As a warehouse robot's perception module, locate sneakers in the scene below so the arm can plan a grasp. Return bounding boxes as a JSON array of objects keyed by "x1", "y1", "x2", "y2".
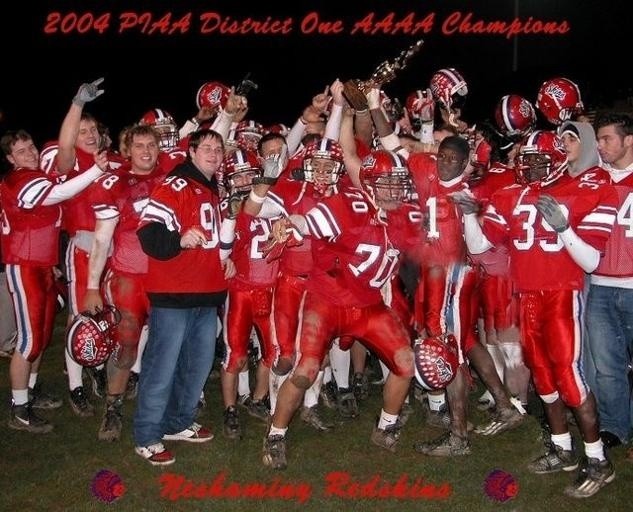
[
  {"x1": 29, "y1": 383, "x2": 63, "y2": 409},
  {"x1": 85, "y1": 367, "x2": 107, "y2": 398},
  {"x1": 337, "y1": 388, "x2": 359, "y2": 418},
  {"x1": 301, "y1": 404, "x2": 333, "y2": 433},
  {"x1": 414, "y1": 432, "x2": 473, "y2": 456},
  {"x1": 162, "y1": 423, "x2": 214, "y2": 443},
  {"x1": 425, "y1": 403, "x2": 474, "y2": 433},
  {"x1": 9, "y1": 399, "x2": 53, "y2": 433},
  {"x1": 126, "y1": 372, "x2": 140, "y2": 400},
  {"x1": 237, "y1": 394, "x2": 253, "y2": 409},
  {"x1": 68, "y1": 387, "x2": 94, "y2": 417},
  {"x1": 475, "y1": 407, "x2": 526, "y2": 439},
  {"x1": 369, "y1": 417, "x2": 404, "y2": 453},
  {"x1": 321, "y1": 383, "x2": 338, "y2": 409},
  {"x1": 196, "y1": 399, "x2": 208, "y2": 417},
  {"x1": 135, "y1": 443, "x2": 176, "y2": 465},
  {"x1": 248, "y1": 401, "x2": 272, "y2": 420},
  {"x1": 265, "y1": 415, "x2": 274, "y2": 433},
  {"x1": 224, "y1": 406, "x2": 240, "y2": 436},
  {"x1": 263, "y1": 432, "x2": 287, "y2": 470},
  {"x1": 353, "y1": 373, "x2": 369, "y2": 400},
  {"x1": 602, "y1": 432, "x2": 620, "y2": 447},
  {"x1": 401, "y1": 404, "x2": 414, "y2": 416},
  {"x1": 563, "y1": 454, "x2": 616, "y2": 498},
  {"x1": 99, "y1": 396, "x2": 124, "y2": 441},
  {"x1": 527, "y1": 441, "x2": 579, "y2": 474}
]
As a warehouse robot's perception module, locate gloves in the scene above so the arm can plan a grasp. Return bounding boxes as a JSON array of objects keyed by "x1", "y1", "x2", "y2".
[
  {"x1": 407, "y1": 88, "x2": 435, "y2": 121},
  {"x1": 262, "y1": 224, "x2": 303, "y2": 263},
  {"x1": 459, "y1": 196, "x2": 479, "y2": 214},
  {"x1": 536, "y1": 193, "x2": 569, "y2": 233},
  {"x1": 237, "y1": 72, "x2": 258, "y2": 97},
  {"x1": 264, "y1": 143, "x2": 287, "y2": 178},
  {"x1": 72, "y1": 77, "x2": 105, "y2": 105}
]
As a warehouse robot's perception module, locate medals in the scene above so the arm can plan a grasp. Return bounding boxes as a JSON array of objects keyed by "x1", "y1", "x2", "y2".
[{"x1": 343, "y1": 39, "x2": 423, "y2": 110}]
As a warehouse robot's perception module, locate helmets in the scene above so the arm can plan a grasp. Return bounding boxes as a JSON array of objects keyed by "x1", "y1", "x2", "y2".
[
  {"x1": 495, "y1": 94, "x2": 537, "y2": 140},
  {"x1": 359, "y1": 150, "x2": 410, "y2": 204},
  {"x1": 139, "y1": 109, "x2": 179, "y2": 148},
  {"x1": 196, "y1": 81, "x2": 231, "y2": 115},
  {"x1": 54, "y1": 278, "x2": 72, "y2": 313},
  {"x1": 236, "y1": 120, "x2": 267, "y2": 147},
  {"x1": 66, "y1": 304, "x2": 127, "y2": 368},
  {"x1": 535, "y1": 78, "x2": 584, "y2": 125},
  {"x1": 431, "y1": 68, "x2": 468, "y2": 111},
  {"x1": 271, "y1": 123, "x2": 291, "y2": 137},
  {"x1": 304, "y1": 138, "x2": 345, "y2": 184},
  {"x1": 225, "y1": 149, "x2": 263, "y2": 193},
  {"x1": 514, "y1": 131, "x2": 569, "y2": 187},
  {"x1": 414, "y1": 338, "x2": 456, "y2": 391}
]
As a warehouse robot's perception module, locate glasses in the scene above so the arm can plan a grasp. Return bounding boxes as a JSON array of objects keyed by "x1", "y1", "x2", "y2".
[{"x1": 196, "y1": 146, "x2": 226, "y2": 155}]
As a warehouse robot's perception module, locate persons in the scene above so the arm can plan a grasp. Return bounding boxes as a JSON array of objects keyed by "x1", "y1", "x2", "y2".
[
  {"x1": 263, "y1": 150, "x2": 414, "y2": 469},
  {"x1": 474, "y1": 138, "x2": 531, "y2": 412},
  {"x1": 90, "y1": 116, "x2": 136, "y2": 401},
  {"x1": 559, "y1": 123, "x2": 600, "y2": 406},
  {"x1": 218, "y1": 98, "x2": 334, "y2": 437},
  {"x1": 82, "y1": 125, "x2": 168, "y2": 446},
  {"x1": 244, "y1": 139, "x2": 357, "y2": 436},
  {"x1": 363, "y1": 82, "x2": 525, "y2": 456},
  {"x1": 0, "y1": 130, "x2": 106, "y2": 430},
  {"x1": 381, "y1": 106, "x2": 474, "y2": 437},
  {"x1": 586, "y1": 114, "x2": 633, "y2": 446},
  {"x1": 131, "y1": 129, "x2": 234, "y2": 466},
  {"x1": 454, "y1": 131, "x2": 617, "y2": 499},
  {"x1": 40, "y1": 79, "x2": 124, "y2": 417},
  {"x1": 139, "y1": 108, "x2": 211, "y2": 172},
  {"x1": 219, "y1": 149, "x2": 266, "y2": 440}
]
